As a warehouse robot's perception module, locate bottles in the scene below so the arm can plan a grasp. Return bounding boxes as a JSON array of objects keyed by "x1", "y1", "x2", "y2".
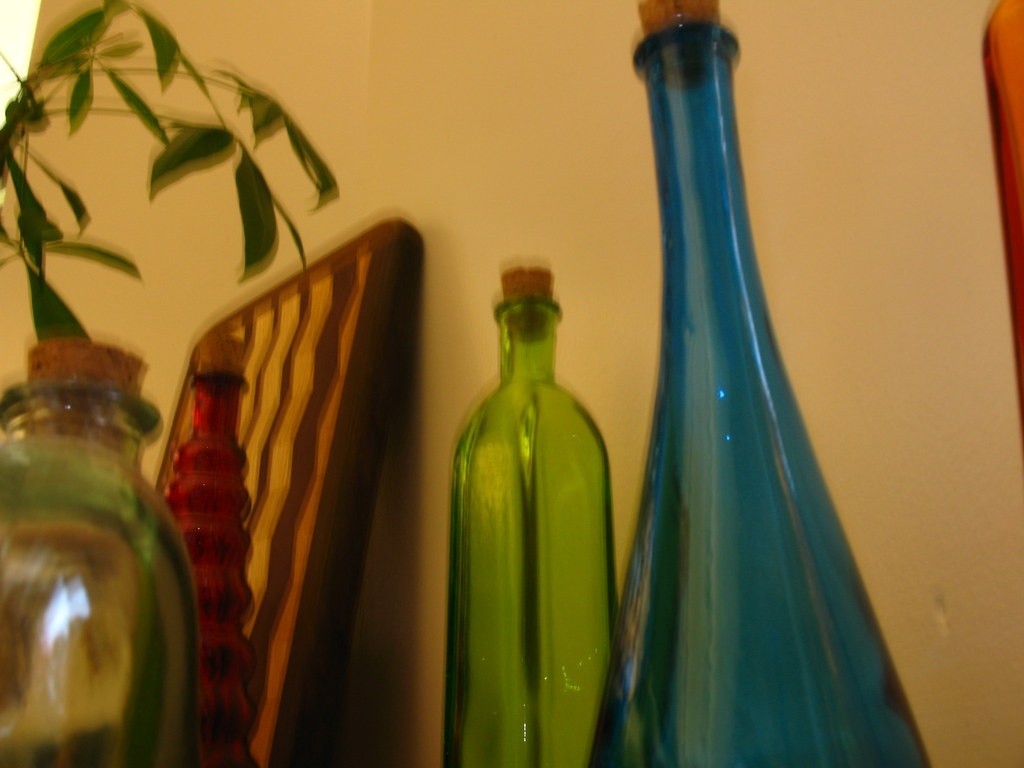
[
  {"x1": 441, "y1": 267, "x2": 614, "y2": 768},
  {"x1": 0, "y1": 341, "x2": 192, "y2": 768},
  {"x1": 593, "y1": 0, "x2": 936, "y2": 768},
  {"x1": 128, "y1": 372, "x2": 255, "y2": 768}
]
